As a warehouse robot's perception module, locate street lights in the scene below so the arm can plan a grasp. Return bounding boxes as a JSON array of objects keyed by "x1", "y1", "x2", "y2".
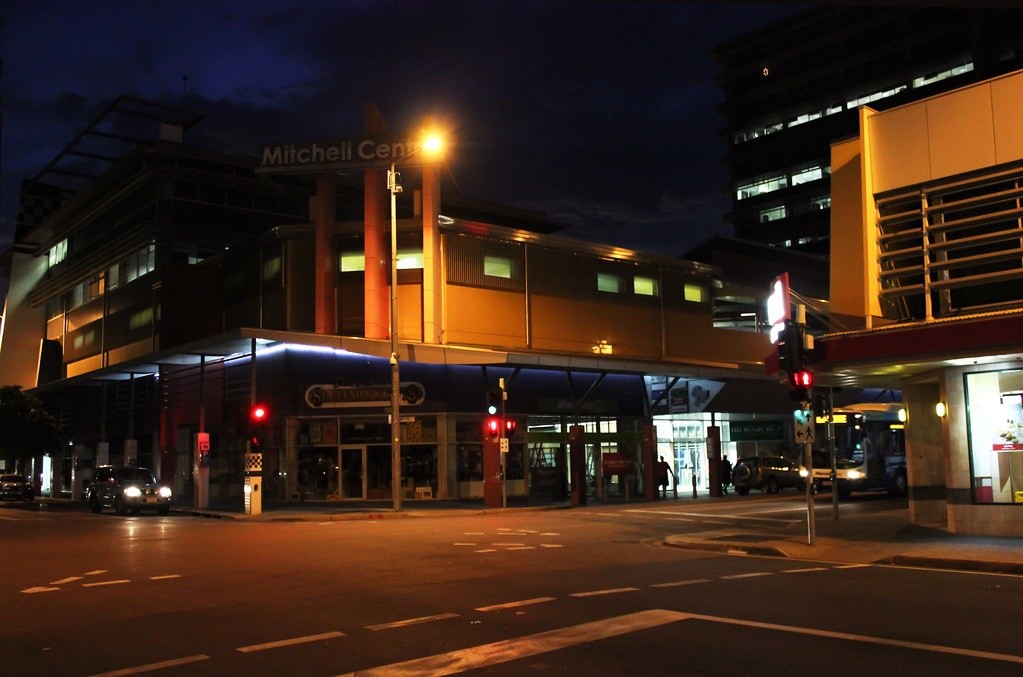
[{"x1": 386, "y1": 132, "x2": 447, "y2": 514}]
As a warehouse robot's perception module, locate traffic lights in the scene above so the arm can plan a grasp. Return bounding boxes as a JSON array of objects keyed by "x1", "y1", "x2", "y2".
[
  {"x1": 489, "y1": 418, "x2": 498, "y2": 437},
  {"x1": 506, "y1": 418, "x2": 517, "y2": 436},
  {"x1": 790, "y1": 366, "x2": 814, "y2": 390},
  {"x1": 252, "y1": 405, "x2": 269, "y2": 424},
  {"x1": 250, "y1": 431, "x2": 265, "y2": 449},
  {"x1": 778, "y1": 324, "x2": 801, "y2": 375}
]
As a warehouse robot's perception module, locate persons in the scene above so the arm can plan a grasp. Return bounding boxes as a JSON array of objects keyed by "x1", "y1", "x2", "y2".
[
  {"x1": 659, "y1": 456, "x2": 675, "y2": 495},
  {"x1": 720, "y1": 455, "x2": 733, "y2": 494}
]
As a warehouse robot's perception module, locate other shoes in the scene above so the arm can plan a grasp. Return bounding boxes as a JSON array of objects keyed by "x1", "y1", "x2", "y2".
[{"x1": 723, "y1": 488, "x2": 728, "y2": 494}]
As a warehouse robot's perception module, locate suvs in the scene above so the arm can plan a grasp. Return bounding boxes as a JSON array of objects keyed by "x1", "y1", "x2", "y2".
[
  {"x1": 85, "y1": 464, "x2": 172, "y2": 517},
  {"x1": 732, "y1": 456, "x2": 805, "y2": 495}
]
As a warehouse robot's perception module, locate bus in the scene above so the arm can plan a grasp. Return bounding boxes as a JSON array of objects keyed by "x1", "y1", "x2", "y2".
[{"x1": 809, "y1": 401, "x2": 909, "y2": 501}]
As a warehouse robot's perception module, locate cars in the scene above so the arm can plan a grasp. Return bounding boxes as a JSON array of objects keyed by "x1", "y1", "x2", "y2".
[{"x1": 0, "y1": 473, "x2": 34, "y2": 501}]
